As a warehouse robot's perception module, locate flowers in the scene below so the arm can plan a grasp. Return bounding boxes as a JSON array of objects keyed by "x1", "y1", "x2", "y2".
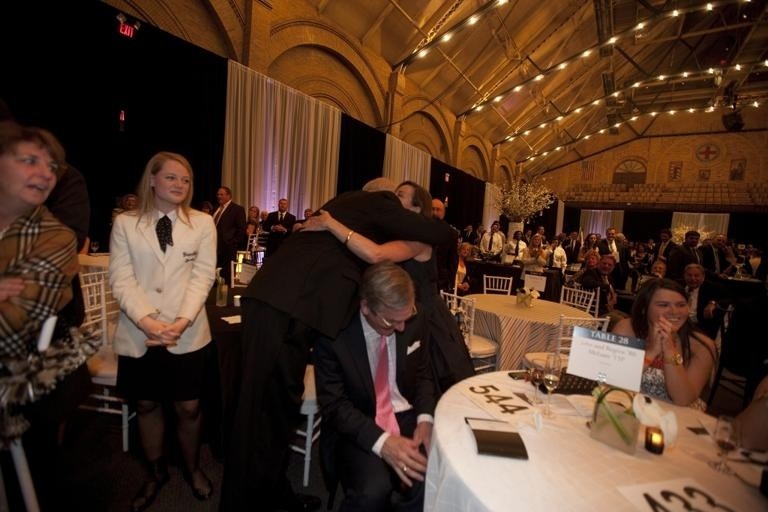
[{"x1": 488, "y1": 180, "x2": 558, "y2": 222}]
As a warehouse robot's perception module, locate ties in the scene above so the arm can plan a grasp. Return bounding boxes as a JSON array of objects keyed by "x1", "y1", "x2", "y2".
[
  {"x1": 488, "y1": 233, "x2": 494, "y2": 250},
  {"x1": 215, "y1": 207, "x2": 222, "y2": 223},
  {"x1": 549, "y1": 251, "x2": 554, "y2": 268},
  {"x1": 373, "y1": 334, "x2": 400, "y2": 435},
  {"x1": 278, "y1": 214, "x2": 284, "y2": 222},
  {"x1": 515, "y1": 240, "x2": 519, "y2": 257}
]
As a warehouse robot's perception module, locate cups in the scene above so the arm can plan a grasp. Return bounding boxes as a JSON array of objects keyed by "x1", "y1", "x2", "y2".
[{"x1": 233, "y1": 295, "x2": 241, "y2": 307}]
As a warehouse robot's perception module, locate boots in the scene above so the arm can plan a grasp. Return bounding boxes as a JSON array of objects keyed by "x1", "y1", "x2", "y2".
[
  {"x1": 131, "y1": 455, "x2": 169, "y2": 511},
  {"x1": 183, "y1": 449, "x2": 212, "y2": 498}
]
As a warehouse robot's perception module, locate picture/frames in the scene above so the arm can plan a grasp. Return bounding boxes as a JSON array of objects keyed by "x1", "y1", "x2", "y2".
[
  {"x1": 728, "y1": 157, "x2": 748, "y2": 182},
  {"x1": 667, "y1": 160, "x2": 684, "y2": 183},
  {"x1": 698, "y1": 168, "x2": 711, "y2": 179}
]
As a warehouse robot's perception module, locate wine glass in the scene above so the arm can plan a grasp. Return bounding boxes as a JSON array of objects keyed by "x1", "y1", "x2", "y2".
[
  {"x1": 529, "y1": 359, "x2": 545, "y2": 405},
  {"x1": 708, "y1": 414, "x2": 740, "y2": 476},
  {"x1": 541, "y1": 352, "x2": 562, "y2": 419},
  {"x1": 91, "y1": 241, "x2": 99, "y2": 256},
  {"x1": 733, "y1": 264, "x2": 743, "y2": 280}
]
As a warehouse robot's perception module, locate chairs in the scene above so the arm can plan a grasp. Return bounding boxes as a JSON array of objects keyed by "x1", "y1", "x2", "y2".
[
  {"x1": 709, "y1": 272, "x2": 766, "y2": 410},
  {"x1": 228, "y1": 231, "x2": 268, "y2": 288},
  {"x1": 74, "y1": 246, "x2": 137, "y2": 451},
  {"x1": 291, "y1": 361, "x2": 320, "y2": 485},
  {"x1": 439, "y1": 245, "x2": 639, "y2": 369}
]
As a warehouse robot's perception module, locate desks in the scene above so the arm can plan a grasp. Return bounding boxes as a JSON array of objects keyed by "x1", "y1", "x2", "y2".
[{"x1": 433, "y1": 368, "x2": 768, "y2": 510}]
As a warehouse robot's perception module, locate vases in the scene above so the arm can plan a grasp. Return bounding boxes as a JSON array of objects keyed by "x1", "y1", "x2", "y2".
[{"x1": 506, "y1": 220, "x2": 525, "y2": 242}]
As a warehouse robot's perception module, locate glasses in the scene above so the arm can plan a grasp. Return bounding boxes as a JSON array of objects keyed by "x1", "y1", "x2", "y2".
[{"x1": 373, "y1": 306, "x2": 417, "y2": 330}]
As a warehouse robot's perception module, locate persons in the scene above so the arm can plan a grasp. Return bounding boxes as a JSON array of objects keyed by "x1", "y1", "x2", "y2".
[
  {"x1": 451, "y1": 220, "x2": 768, "y2": 414},
  {"x1": 197, "y1": 186, "x2": 313, "y2": 286},
  {"x1": 107, "y1": 152, "x2": 219, "y2": 500},
  {"x1": 1, "y1": 102, "x2": 91, "y2": 346},
  {"x1": 0, "y1": 121, "x2": 81, "y2": 362},
  {"x1": 239, "y1": 177, "x2": 460, "y2": 512}
]
